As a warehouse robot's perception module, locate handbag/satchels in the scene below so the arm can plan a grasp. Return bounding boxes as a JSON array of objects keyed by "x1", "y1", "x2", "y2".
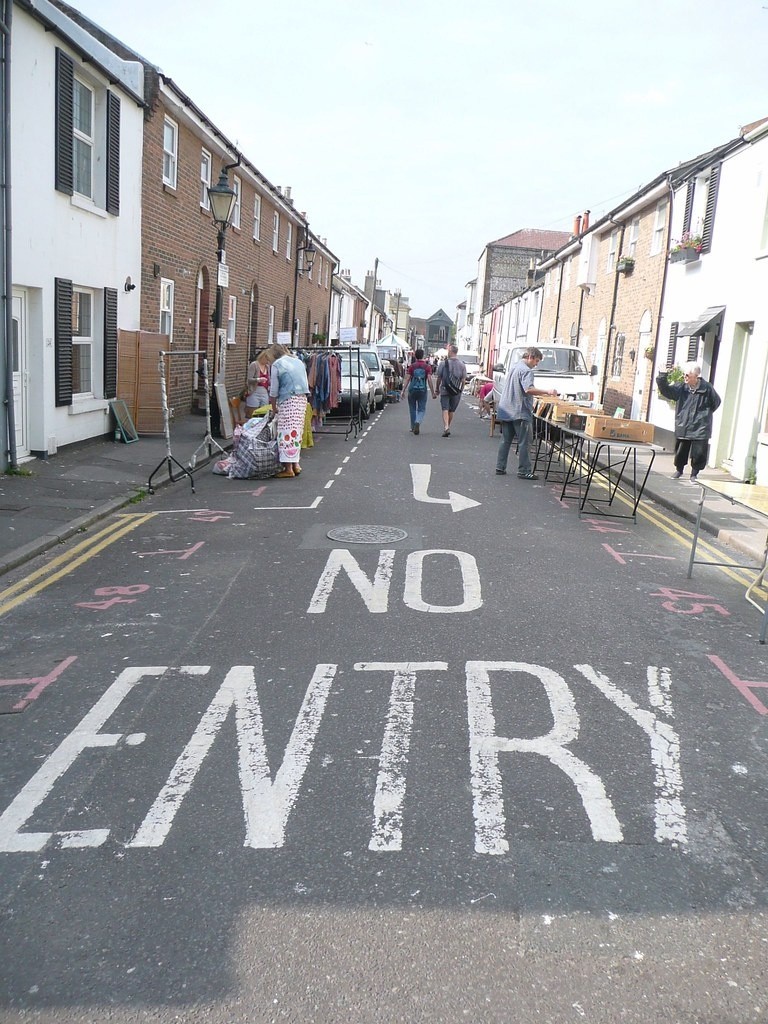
[
  {"x1": 445, "y1": 374, "x2": 461, "y2": 395},
  {"x1": 230, "y1": 432, "x2": 282, "y2": 478}
]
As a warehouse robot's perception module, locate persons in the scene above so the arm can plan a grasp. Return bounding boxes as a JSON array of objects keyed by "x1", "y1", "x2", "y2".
[
  {"x1": 426, "y1": 354, "x2": 448, "y2": 375},
  {"x1": 656, "y1": 363, "x2": 722, "y2": 484},
  {"x1": 246, "y1": 351, "x2": 271, "y2": 419},
  {"x1": 268, "y1": 343, "x2": 310, "y2": 477},
  {"x1": 403, "y1": 352, "x2": 416, "y2": 372},
  {"x1": 496, "y1": 347, "x2": 557, "y2": 479},
  {"x1": 400, "y1": 350, "x2": 437, "y2": 435},
  {"x1": 435, "y1": 346, "x2": 466, "y2": 437}
]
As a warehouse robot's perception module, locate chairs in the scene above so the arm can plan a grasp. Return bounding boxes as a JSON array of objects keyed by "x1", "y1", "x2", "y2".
[
  {"x1": 229, "y1": 397, "x2": 249, "y2": 431},
  {"x1": 544, "y1": 357, "x2": 555, "y2": 368}
]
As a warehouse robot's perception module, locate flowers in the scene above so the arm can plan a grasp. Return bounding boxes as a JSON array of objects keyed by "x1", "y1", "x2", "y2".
[
  {"x1": 667, "y1": 234, "x2": 703, "y2": 253},
  {"x1": 645, "y1": 347, "x2": 654, "y2": 352},
  {"x1": 658, "y1": 362, "x2": 686, "y2": 405},
  {"x1": 615, "y1": 255, "x2": 634, "y2": 264}
]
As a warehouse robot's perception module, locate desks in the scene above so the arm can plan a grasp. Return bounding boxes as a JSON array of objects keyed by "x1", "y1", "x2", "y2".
[
  {"x1": 473, "y1": 378, "x2": 493, "y2": 398},
  {"x1": 511, "y1": 411, "x2": 665, "y2": 525},
  {"x1": 251, "y1": 403, "x2": 314, "y2": 449},
  {"x1": 686, "y1": 476, "x2": 768, "y2": 645}
]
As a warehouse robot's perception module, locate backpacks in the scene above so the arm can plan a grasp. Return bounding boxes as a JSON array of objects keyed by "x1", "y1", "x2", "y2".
[{"x1": 409, "y1": 362, "x2": 428, "y2": 393}]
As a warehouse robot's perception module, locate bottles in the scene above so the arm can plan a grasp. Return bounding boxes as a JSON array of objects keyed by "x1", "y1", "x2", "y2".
[
  {"x1": 115, "y1": 423, "x2": 121, "y2": 441},
  {"x1": 613, "y1": 407, "x2": 625, "y2": 419},
  {"x1": 481, "y1": 371, "x2": 484, "y2": 377}
]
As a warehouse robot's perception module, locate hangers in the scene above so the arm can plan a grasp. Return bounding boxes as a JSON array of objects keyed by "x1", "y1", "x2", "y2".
[{"x1": 331, "y1": 347, "x2": 337, "y2": 356}]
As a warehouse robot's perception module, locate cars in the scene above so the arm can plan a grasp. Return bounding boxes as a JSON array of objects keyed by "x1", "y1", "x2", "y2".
[{"x1": 326, "y1": 344, "x2": 407, "y2": 420}]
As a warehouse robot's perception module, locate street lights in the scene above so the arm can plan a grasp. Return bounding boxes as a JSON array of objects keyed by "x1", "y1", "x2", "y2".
[
  {"x1": 291, "y1": 239, "x2": 317, "y2": 347},
  {"x1": 205, "y1": 167, "x2": 239, "y2": 439}
]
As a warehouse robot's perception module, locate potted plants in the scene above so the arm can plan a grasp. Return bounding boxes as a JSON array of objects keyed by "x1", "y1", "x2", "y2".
[{"x1": 312, "y1": 333, "x2": 326, "y2": 344}]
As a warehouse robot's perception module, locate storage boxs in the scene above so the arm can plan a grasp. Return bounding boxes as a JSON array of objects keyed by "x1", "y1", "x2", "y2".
[{"x1": 534, "y1": 395, "x2": 655, "y2": 442}]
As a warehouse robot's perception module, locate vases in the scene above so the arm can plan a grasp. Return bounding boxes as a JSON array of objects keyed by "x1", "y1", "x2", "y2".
[
  {"x1": 645, "y1": 352, "x2": 653, "y2": 360},
  {"x1": 617, "y1": 263, "x2": 633, "y2": 272},
  {"x1": 670, "y1": 248, "x2": 699, "y2": 265}
]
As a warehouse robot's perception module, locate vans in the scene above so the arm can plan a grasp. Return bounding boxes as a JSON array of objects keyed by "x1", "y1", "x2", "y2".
[{"x1": 493, "y1": 343, "x2": 597, "y2": 432}]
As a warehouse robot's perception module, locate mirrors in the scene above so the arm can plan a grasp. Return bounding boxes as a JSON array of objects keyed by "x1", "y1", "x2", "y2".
[{"x1": 110, "y1": 400, "x2": 139, "y2": 444}]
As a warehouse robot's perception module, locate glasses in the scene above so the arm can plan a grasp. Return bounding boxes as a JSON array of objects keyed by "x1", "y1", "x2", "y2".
[{"x1": 683, "y1": 373, "x2": 693, "y2": 377}]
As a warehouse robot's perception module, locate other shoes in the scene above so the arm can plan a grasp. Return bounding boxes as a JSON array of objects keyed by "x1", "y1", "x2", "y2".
[
  {"x1": 496, "y1": 468, "x2": 507, "y2": 474},
  {"x1": 274, "y1": 471, "x2": 296, "y2": 478},
  {"x1": 442, "y1": 428, "x2": 450, "y2": 438},
  {"x1": 484, "y1": 414, "x2": 490, "y2": 419},
  {"x1": 690, "y1": 474, "x2": 699, "y2": 485},
  {"x1": 517, "y1": 470, "x2": 538, "y2": 480},
  {"x1": 409, "y1": 421, "x2": 420, "y2": 434},
  {"x1": 671, "y1": 470, "x2": 683, "y2": 479},
  {"x1": 294, "y1": 465, "x2": 302, "y2": 476}
]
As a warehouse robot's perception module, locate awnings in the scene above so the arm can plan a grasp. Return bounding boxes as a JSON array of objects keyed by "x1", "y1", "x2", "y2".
[{"x1": 676, "y1": 306, "x2": 725, "y2": 336}]
